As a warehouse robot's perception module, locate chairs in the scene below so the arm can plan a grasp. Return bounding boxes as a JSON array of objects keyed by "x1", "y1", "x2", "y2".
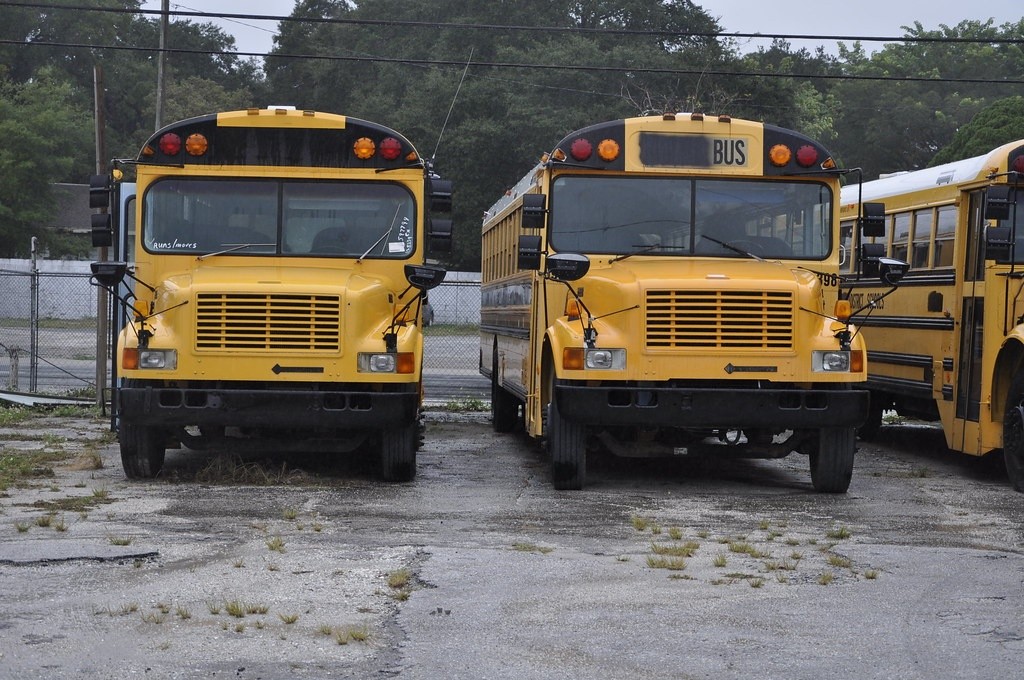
[
  {"x1": 714, "y1": 235, "x2": 793, "y2": 256},
  {"x1": 179, "y1": 223, "x2": 274, "y2": 251},
  {"x1": 312, "y1": 227, "x2": 398, "y2": 252}
]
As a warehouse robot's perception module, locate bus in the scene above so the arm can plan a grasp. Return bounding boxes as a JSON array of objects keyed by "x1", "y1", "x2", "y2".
[
  {"x1": 478, "y1": 113, "x2": 911, "y2": 491},
  {"x1": 840, "y1": 139, "x2": 1024, "y2": 493},
  {"x1": 87, "y1": 106, "x2": 457, "y2": 482}
]
